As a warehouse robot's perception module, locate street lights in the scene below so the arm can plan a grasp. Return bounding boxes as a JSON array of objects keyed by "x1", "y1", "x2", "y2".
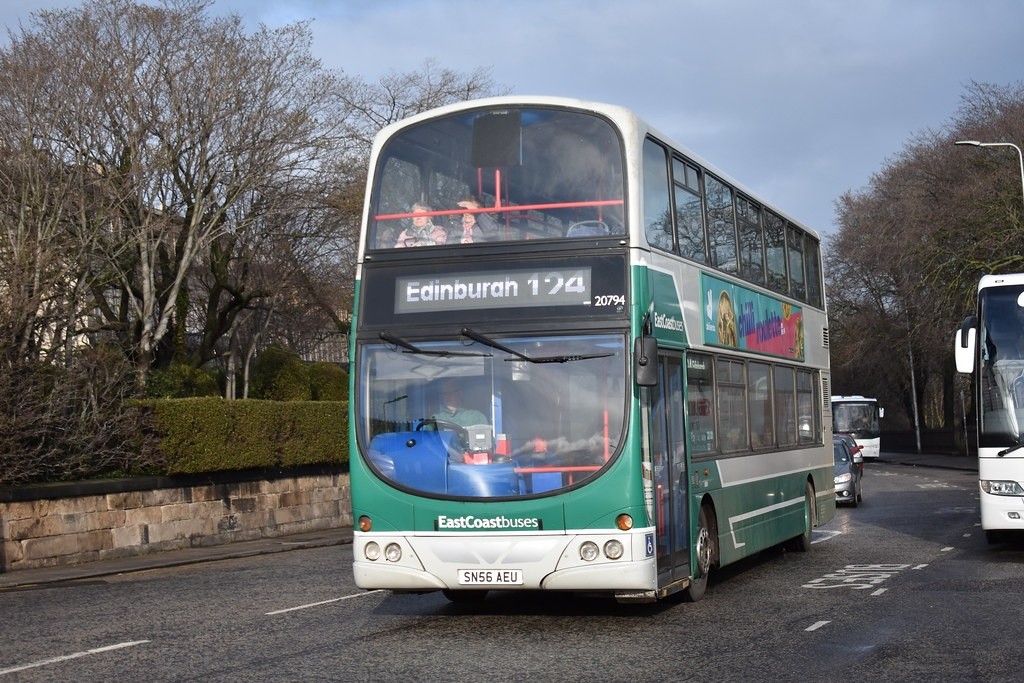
[{"x1": 954, "y1": 141, "x2": 1024, "y2": 197}]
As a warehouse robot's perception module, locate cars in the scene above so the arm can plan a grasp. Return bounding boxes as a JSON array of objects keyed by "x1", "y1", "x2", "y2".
[
  {"x1": 833, "y1": 434, "x2": 864, "y2": 472},
  {"x1": 833, "y1": 439, "x2": 864, "y2": 508}
]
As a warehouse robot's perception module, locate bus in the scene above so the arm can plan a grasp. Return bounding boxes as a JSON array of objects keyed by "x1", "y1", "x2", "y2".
[
  {"x1": 954, "y1": 272, "x2": 1024, "y2": 550},
  {"x1": 349, "y1": 95, "x2": 836, "y2": 602},
  {"x1": 830, "y1": 395, "x2": 884, "y2": 462}
]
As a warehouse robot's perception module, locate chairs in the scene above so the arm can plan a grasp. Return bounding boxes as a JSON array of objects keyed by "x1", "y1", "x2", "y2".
[{"x1": 566, "y1": 220, "x2": 609, "y2": 237}]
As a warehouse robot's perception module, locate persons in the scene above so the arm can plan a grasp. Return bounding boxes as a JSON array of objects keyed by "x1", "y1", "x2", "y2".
[
  {"x1": 446, "y1": 200, "x2": 499, "y2": 244},
  {"x1": 393, "y1": 202, "x2": 449, "y2": 248},
  {"x1": 717, "y1": 290, "x2": 738, "y2": 347},
  {"x1": 424, "y1": 380, "x2": 489, "y2": 431}
]
